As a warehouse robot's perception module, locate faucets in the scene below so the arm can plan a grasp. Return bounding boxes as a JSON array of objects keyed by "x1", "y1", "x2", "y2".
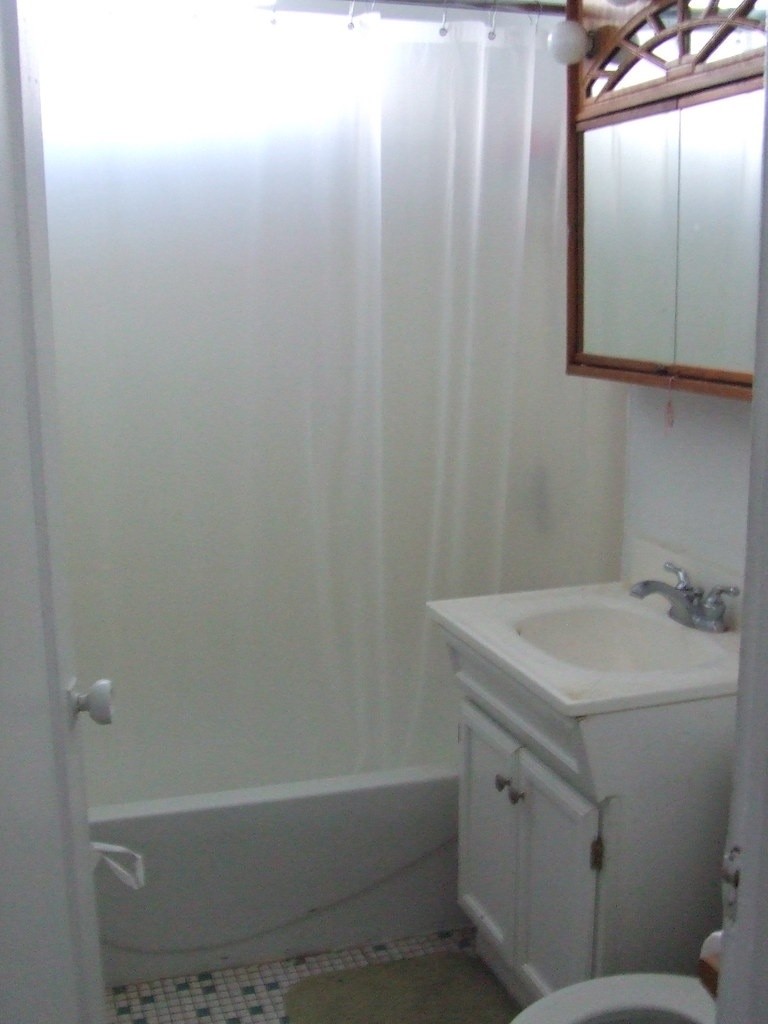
[{"x1": 627, "y1": 579, "x2": 700, "y2": 626}]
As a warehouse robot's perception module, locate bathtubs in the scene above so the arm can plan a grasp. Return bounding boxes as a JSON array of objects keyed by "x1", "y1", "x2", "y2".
[{"x1": 85, "y1": 759, "x2": 462, "y2": 990}]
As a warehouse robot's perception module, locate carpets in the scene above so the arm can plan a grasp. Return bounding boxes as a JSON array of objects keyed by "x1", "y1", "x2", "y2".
[{"x1": 286, "y1": 948, "x2": 520, "y2": 1024}]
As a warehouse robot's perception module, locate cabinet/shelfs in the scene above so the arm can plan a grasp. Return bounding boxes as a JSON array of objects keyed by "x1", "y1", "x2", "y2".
[{"x1": 435, "y1": 622, "x2": 740, "y2": 1012}]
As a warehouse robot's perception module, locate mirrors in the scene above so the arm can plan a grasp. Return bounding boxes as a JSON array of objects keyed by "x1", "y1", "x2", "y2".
[{"x1": 558, "y1": 0, "x2": 768, "y2": 398}]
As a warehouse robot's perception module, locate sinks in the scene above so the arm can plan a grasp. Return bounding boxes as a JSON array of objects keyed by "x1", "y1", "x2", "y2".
[{"x1": 511, "y1": 597, "x2": 727, "y2": 676}]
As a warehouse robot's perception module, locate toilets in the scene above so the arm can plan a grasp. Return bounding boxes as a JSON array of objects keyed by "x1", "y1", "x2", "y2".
[{"x1": 511, "y1": 970, "x2": 720, "y2": 1024}]
